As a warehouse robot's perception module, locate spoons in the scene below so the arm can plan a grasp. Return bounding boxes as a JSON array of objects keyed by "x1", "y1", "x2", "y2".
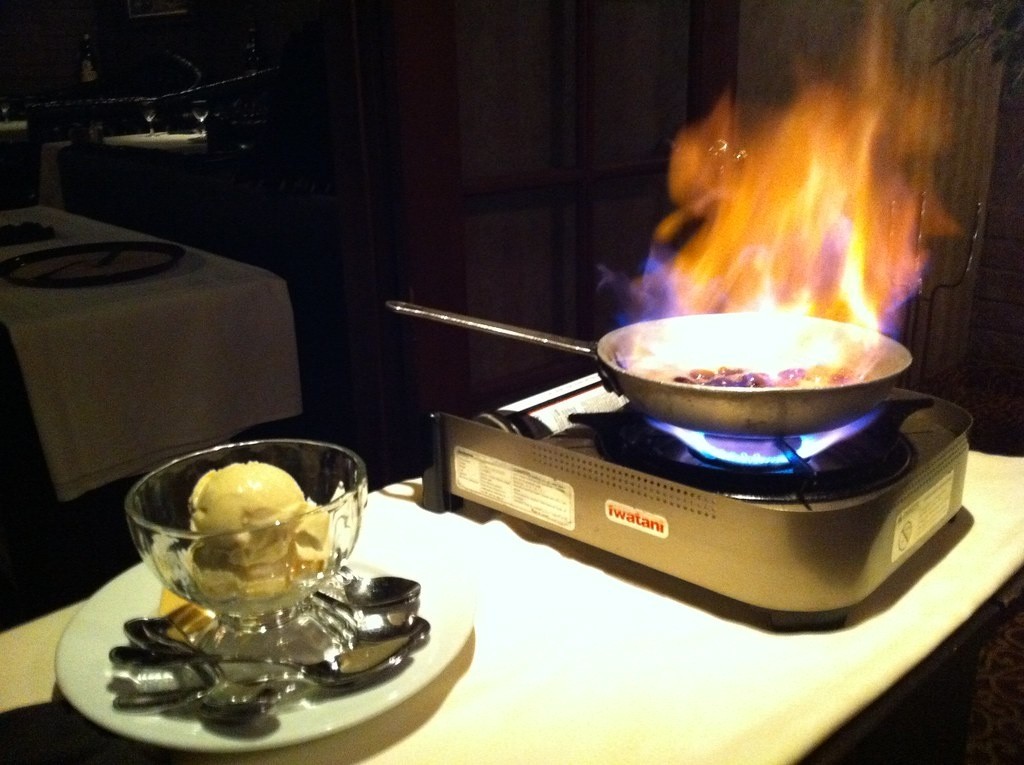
[
  {"x1": 312, "y1": 590, "x2": 430, "y2": 646},
  {"x1": 124, "y1": 617, "x2": 282, "y2": 725},
  {"x1": 109, "y1": 636, "x2": 415, "y2": 691},
  {"x1": 33, "y1": 250, "x2": 124, "y2": 279},
  {"x1": 336, "y1": 565, "x2": 421, "y2": 608}
]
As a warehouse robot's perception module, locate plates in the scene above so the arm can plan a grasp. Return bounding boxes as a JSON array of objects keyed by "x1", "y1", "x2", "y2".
[
  {"x1": 55, "y1": 560, "x2": 474, "y2": 752},
  {"x1": 0, "y1": 239, "x2": 186, "y2": 289}
]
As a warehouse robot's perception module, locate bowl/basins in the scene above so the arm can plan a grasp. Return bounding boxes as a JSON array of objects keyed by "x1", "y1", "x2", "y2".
[{"x1": 126, "y1": 439, "x2": 367, "y2": 662}]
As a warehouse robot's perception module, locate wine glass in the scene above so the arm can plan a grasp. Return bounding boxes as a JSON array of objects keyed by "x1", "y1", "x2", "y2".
[
  {"x1": 190, "y1": 101, "x2": 209, "y2": 145},
  {"x1": 141, "y1": 100, "x2": 159, "y2": 138}
]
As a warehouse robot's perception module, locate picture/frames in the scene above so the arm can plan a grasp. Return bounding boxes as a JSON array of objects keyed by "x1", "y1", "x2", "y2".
[{"x1": 127, "y1": 0, "x2": 189, "y2": 20}]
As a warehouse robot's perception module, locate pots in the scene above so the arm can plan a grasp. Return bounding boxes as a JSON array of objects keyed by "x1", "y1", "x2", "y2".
[{"x1": 385, "y1": 298, "x2": 913, "y2": 435}]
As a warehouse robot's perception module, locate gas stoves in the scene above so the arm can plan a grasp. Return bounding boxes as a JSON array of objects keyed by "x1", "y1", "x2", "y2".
[{"x1": 422, "y1": 372, "x2": 975, "y2": 632}]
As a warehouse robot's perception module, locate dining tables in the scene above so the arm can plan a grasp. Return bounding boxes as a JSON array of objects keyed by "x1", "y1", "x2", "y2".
[
  {"x1": 0, "y1": 205, "x2": 303, "y2": 503},
  {"x1": 0, "y1": 120, "x2": 27, "y2": 147},
  {"x1": 0, "y1": 449, "x2": 1024, "y2": 764},
  {"x1": 38, "y1": 132, "x2": 207, "y2": 213}
]
